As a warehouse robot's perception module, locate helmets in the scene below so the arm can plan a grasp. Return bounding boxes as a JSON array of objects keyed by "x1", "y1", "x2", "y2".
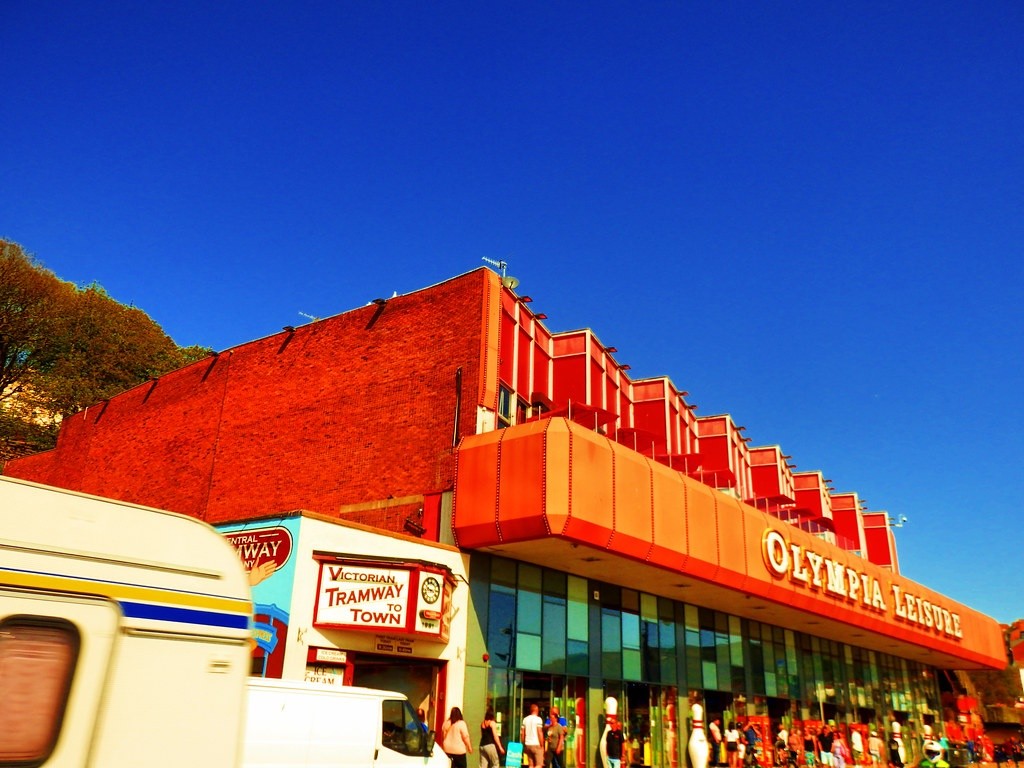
[{"x1": 923, "y1": 741, "x2": 944, "y2": 763}]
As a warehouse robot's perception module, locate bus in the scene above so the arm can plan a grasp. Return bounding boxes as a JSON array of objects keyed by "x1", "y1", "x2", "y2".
[{"x1": 0, "y1": 471, "x2": 261, "y2": 768}]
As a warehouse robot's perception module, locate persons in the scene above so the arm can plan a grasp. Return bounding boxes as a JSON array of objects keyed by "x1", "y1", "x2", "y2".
[
  {"x1": 544, "y1": 706, "x2": 567, "y2": 768},
  {"x1": 479, "y1": 709, "x2": 505, "y2": 768},
  {"x1": 994, "y1": 737, "x2": 1024, "y2": 763},
  {"x1": 917, "y1": 740, "x2": 950, "y2": 768},
  {"x1": 964, "y1": 737, "x2": 983, "y2": 763},
  {"x1": 775, "y1": 724, "x2": 904, "y2": 768},
  {"x1": 407, "y1": 709, "x2": 428, "y2": 732},
  {"x1": 522, "y1": 704, "x2": 544, "y2": 768},
  {"x1": 606, "y1": 719, "x2": 625, "y2": 768},
  {"x1": 709, "y1": 717, "x2": 762, "y2": 768},
  {"x1": 442, "y1": 707, "x2": 473, "y2": 768}
]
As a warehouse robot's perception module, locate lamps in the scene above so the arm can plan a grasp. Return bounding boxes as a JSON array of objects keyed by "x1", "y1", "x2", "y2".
[
  {"x1": 103, "y1": 397, "x2": 111, "y2": 402},
  {"x1": 606, "y1": 346, "x2": 617, "y2": 352},
  {"x1": 206, "y1": 351, "x2": 219, "y2": 357},
  {"x1": 520, "y1": 296, "x2": 532, "y2": 302},
  {"x1": 680, "y1": 391, "x2": 906, "y2": 528},
  {"x1": 372, "y1": 298, "x2": 387, "y2": 305},
  {"x1": 537, "y1": 314, "x2": 547, "y2": 320},
  {"x1": 283, "y1": 326, "x2": 293, "y2": 331},
  {"x1": 621, "y1": 365, "x2": 631, "y2": 370},
  {"x1": 146, "y1": 376, "x2": 160, "y2": 381}
]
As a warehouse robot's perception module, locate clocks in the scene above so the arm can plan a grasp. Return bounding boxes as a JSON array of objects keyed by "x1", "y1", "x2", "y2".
[{"x1": 422, "y1": 577, "x2": 440, "y2": 604}]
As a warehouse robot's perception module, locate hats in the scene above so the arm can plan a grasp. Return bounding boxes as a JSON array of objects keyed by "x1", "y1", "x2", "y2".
[{"x1": 871, "y1": 731, "x2": 877, "y2": 736}]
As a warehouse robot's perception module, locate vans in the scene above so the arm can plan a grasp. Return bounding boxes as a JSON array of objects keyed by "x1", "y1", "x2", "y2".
[{"x1": 244, "y1": 676, "x2": 454, "y2": 768}]
